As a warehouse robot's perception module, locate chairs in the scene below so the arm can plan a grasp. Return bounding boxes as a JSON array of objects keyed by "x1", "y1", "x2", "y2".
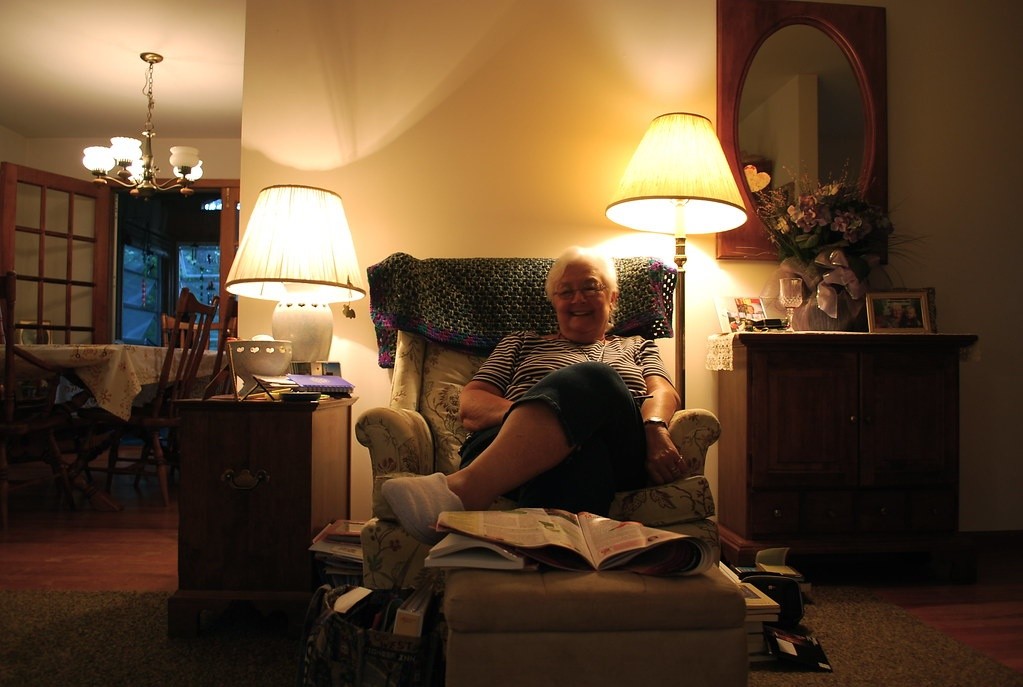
[
  {"x1": 61, "y1": 286, "x2": 219, "y2": 508},
  {"x1": 0, "y1": 308, "x2": 120, "y2": 514},
  {"x1": 160, "y1": 311, "x2": 238, "y2": 352},
  {"x1": 0, "y1": 269, "x2": 79, "y2": 527}
]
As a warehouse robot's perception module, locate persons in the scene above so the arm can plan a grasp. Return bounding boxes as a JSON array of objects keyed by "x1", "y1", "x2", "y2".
[
  {"x1": 382, "y1": 246, "x2": 688, "y2": 547},
  {"x1": 886, "y1": 301, "x2": 917, "y2": 328}
]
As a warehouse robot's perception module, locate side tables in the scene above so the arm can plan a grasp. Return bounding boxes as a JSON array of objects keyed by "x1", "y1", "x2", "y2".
[
  {"x1": 167, "y1": 392, "x2": 356, "y2": 639},
  {"x1": 715, "y1": 330, "x2": 980, "y2": 591}
]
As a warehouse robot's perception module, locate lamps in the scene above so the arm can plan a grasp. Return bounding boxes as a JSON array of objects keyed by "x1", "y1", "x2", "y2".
[
  {"x1": 605, "y1": 110, "x2": 749, "y2": 412},
  {"x1": 82, "y1": 52, "x2": 204, "y2": 199},
  {"x1": 221, "y1": 183, "x2": 367, "y2": 375}
]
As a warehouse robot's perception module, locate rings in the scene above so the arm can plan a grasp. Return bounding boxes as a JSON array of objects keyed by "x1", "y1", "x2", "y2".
[
  {"x1": 671, "y1": 465, "x2": 679, "y2": 474},
  {"x1": 672, "y1": 457, "x2": 683, "y2": 464}
]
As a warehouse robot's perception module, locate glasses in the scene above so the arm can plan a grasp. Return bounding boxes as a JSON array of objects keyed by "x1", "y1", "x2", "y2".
[{"x1": 553, "y1": 282, "x2": 607, "y2": 300}]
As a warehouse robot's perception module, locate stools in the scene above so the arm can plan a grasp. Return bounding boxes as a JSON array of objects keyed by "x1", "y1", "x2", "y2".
[{"x1": 442, "y1": 569, "x2": 751, "y2": 687}]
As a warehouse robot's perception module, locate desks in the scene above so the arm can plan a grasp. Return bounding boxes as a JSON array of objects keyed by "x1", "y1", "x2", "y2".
[{"x1": 0, "y1": 342, "x2": 229, "y2": 512}]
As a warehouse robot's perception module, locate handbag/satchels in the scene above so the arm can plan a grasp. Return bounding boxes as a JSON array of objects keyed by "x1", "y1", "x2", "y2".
[
  {"x1": 738, "y1": 571, "x2": 804, "y2": 630},
  {"x1": 302, "y1": 590, "x2": 445, "y2": 687}
]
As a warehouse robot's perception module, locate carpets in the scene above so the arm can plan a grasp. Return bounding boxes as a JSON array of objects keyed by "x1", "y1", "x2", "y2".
[{"x1": 0, "y1": 584, "x2": 1023, "y2": 687}]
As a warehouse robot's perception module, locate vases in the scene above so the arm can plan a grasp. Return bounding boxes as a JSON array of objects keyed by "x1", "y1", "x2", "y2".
[{"x1": 790, "y1": 274, "x2": 870, "y2": 330}]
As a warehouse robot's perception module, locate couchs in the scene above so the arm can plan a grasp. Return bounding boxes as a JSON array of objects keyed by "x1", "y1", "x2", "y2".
[{"x1": 353, "y1": 252, "x2": 722, "y2": 579}]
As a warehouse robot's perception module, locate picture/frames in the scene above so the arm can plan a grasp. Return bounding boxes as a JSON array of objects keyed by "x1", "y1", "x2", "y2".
[{"x1": 865, "y1": 290, "x2": 933, "y2": 334}]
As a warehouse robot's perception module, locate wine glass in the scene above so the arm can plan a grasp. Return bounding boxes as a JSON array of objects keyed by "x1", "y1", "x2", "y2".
[{"x1": 780, "y1": 277, "x2": 803, "y2": 332}]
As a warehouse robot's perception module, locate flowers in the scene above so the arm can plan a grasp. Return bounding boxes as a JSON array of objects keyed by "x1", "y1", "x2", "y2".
[{"x1": 750, "y1": 152, "x2": 935, "y2": 292}]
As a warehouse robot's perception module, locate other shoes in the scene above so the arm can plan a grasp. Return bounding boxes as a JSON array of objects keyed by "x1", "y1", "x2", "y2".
[{"x1": 379, "y1": 471, "x2": 464, "y2": 543}]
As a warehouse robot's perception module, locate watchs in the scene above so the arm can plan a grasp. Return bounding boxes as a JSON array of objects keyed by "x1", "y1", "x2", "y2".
[{"x1": 643, "y1": 416, "x2": 668, "y2": 429}]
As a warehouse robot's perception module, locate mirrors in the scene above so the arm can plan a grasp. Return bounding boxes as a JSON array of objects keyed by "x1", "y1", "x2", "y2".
[{"x1": 716, "y1": 0, "x2": 889, "y2": 263}]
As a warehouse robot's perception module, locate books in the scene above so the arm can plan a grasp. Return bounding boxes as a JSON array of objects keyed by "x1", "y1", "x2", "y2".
[
  {"x1": 250, "y1": 361, "x2": 355, "y2": 400},
  {"x1": 336, "y1": 581, "x2": 434, "y2": 636},
  {"x1": 742, "y1": 547, "x2": 832, "y2": 671},
  {"x1": 425, "y1": 508, "x2": 714, "y2": 577},
  {"x1": 307, "y1": 521, "x2": 369, "y2": 575}
]
